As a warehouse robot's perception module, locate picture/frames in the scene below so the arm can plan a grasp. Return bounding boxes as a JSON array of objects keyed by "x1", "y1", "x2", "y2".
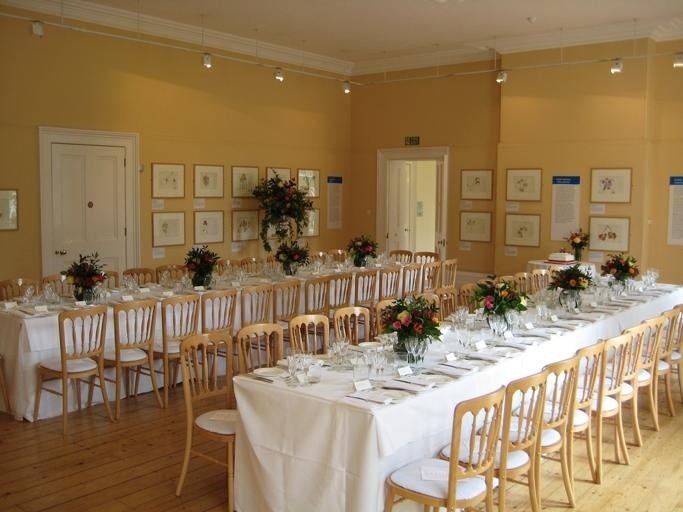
[
  {"x1": 459, "y1": 167, "x2": 633, "y2": 253},
  {"x1": 0, "y1": 188, "x2": 20, "y2": 231},
  {"x1": 151, "y1": 161, "x2": 321, "y2": 248}
]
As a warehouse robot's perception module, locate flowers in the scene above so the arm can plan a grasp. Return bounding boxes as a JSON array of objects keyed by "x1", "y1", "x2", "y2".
[{"x1": 345, "y1": 233, "x2": 379, "y2": 261}]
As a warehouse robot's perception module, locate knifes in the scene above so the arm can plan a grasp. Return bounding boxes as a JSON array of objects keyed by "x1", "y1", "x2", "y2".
[{"x1": 391, "y1": 378, "x2": 427, "y2": 387}]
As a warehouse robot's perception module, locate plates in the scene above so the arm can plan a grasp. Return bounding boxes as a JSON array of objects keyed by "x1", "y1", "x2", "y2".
[
  {"x1": 357, "y1": 341, "x2": 384, "y2": 350},
  {"x1": 253, "y1": 366, "x2": 284, "y2": 377},
  {"x1": 424, "y1": 374, "x2": 451, "y2": 384},
  {"x1": 381, "y1": 389, "x2": 410, "y2": 404},
  {"x1": 467, "y1": 359, "x2": 491, "y2": 369}
]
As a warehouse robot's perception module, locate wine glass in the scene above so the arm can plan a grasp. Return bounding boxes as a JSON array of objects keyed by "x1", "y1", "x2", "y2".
[
  {"x1": 289, "y1": 262, "x2": 660, "y2": 387},
  {"x1": 16, "y1": 238, "x2": 384, "y2": 305}
]
